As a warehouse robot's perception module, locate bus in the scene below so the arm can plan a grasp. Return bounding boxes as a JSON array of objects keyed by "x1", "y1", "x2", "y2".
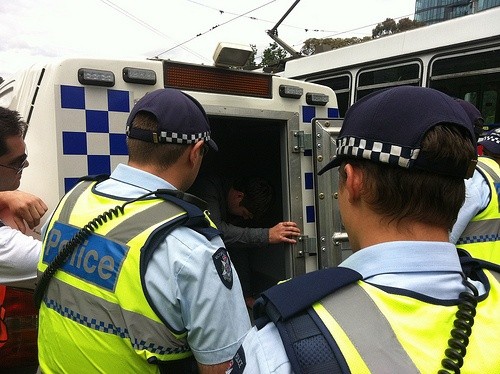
[{"x1": 246, "y1": 0, "x2": 500, "y2": 129}]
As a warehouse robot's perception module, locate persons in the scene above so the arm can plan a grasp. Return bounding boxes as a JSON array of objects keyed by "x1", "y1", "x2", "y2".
[
  {"x1": 227, "y1": 86, "x2": 500, "y2": 374},
  {"x1": 0, "y1": 106, "x2": 48, "y2": 348},
  {"x1": 449, "y1": 98, "x2": 500, "y2": 265},
  {"x1": 191, "y1": 166, "x2": 300, "y2": 309},
  {"x1": 477, "y1": 127, "x2": 500, "y2": 166},
  {"x1": 36, "y1": 88, "x2": 252, "y2": 374}
]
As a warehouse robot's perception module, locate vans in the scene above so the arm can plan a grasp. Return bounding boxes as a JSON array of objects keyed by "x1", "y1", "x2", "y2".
[{"x1": 0, "y1": 41, "x2": 354, "y2": 373}]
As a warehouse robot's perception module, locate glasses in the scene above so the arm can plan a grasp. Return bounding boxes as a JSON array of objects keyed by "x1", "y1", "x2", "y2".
[{"x1": 0, "y1": 155, "x2": 28, "y2": 174}]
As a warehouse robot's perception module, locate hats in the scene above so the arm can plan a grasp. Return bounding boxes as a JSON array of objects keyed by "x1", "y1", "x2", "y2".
[
  {"x1": 126, "y1": 88, "x2": 218, "y2": 152},
  {"x1": 315, "y1": 85, "x2": 478, "y2": 177},
  {"x1": 478, "y1": 127, "x2": 500, "y2": 154},
  {"x1": 452, "y1": 95, "x2": 484, "y2": 133}
]
